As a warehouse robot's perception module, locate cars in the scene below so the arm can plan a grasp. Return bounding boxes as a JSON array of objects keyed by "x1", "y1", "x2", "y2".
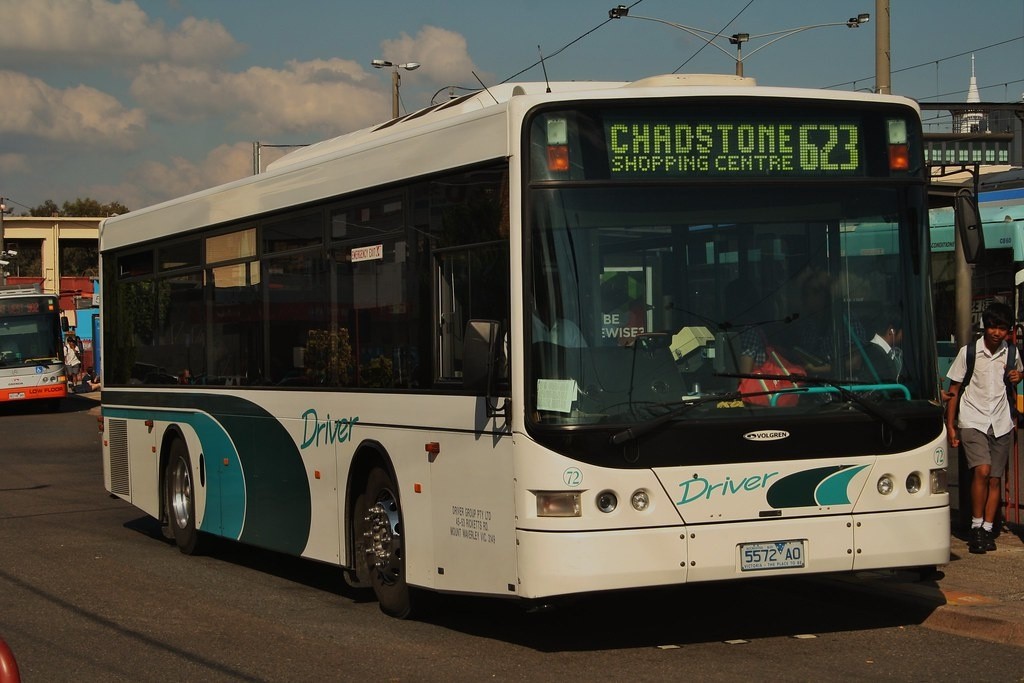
[
  {"x1": 144, "y1": 373, "x2": 179, "y2": 384},
  {"x1": 252, "y1": 366, "x2": 331, "y2": 387}
]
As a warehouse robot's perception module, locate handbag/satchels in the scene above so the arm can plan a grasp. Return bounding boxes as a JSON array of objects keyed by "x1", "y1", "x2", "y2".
[{"x1": 738, "y1": 318, "x2": 807, "y2": 408}]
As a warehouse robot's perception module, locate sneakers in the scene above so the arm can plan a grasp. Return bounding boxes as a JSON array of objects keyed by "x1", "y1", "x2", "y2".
[{"x1": 966, "y1": 526, "x2": 997, "y2": 554}]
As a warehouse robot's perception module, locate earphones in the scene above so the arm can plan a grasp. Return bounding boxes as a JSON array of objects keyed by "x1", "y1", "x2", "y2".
[{"x1": 891, "y1": 329, "x2": 894, "y2": 334}]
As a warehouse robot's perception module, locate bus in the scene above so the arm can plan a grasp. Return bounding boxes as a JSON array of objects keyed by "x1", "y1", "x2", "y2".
[
  {"x1": 0, "y1": 288, "x2": 69, "y2": 401},
  {"x1": 97, "y1": 74, "x2": 985, "y2": 620},
  {"x1": 658, "y1": 205, "x2": 1024, "y2": 415}
]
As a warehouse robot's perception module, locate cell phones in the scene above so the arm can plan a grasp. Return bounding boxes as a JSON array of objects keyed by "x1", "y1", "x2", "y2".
[{"x1": 946, "y1": 392, "x2": 955, "y2": 397}]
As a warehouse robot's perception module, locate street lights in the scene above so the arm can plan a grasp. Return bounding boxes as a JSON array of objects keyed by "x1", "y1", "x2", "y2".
[
  {"x1": 609, "y1": 5, "x2": 870, "y2": 78},
  {"x1": 345, "y1": 254, "x2": 360, "y2": 388},
  {"x1": 371, "y1": 59, "x2": 421, "y2": 119},
  {"x1": 663, "y1": 301, "x2": 799, "y2": 374}
]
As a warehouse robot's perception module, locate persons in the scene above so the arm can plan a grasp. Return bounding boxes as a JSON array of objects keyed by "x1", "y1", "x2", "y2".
[
  {"x1": 75, "y1": 336, "x2": 84, "y2": 373},
  {"x1": 68, "y1": 368, "x2": 101, "y2": 393},
  {"x1": 64, "y1": 336, "x2": 81, "y2": 385},
  {"x1": 504, "y1": 273, "x2": 863, "y2": 376},
  {"x1": 862, "y1": 312, "x2": 952, "y2": 400},
  {"x1": 947, "y1": 303, "x2": 1024, "y2": 553}
]
